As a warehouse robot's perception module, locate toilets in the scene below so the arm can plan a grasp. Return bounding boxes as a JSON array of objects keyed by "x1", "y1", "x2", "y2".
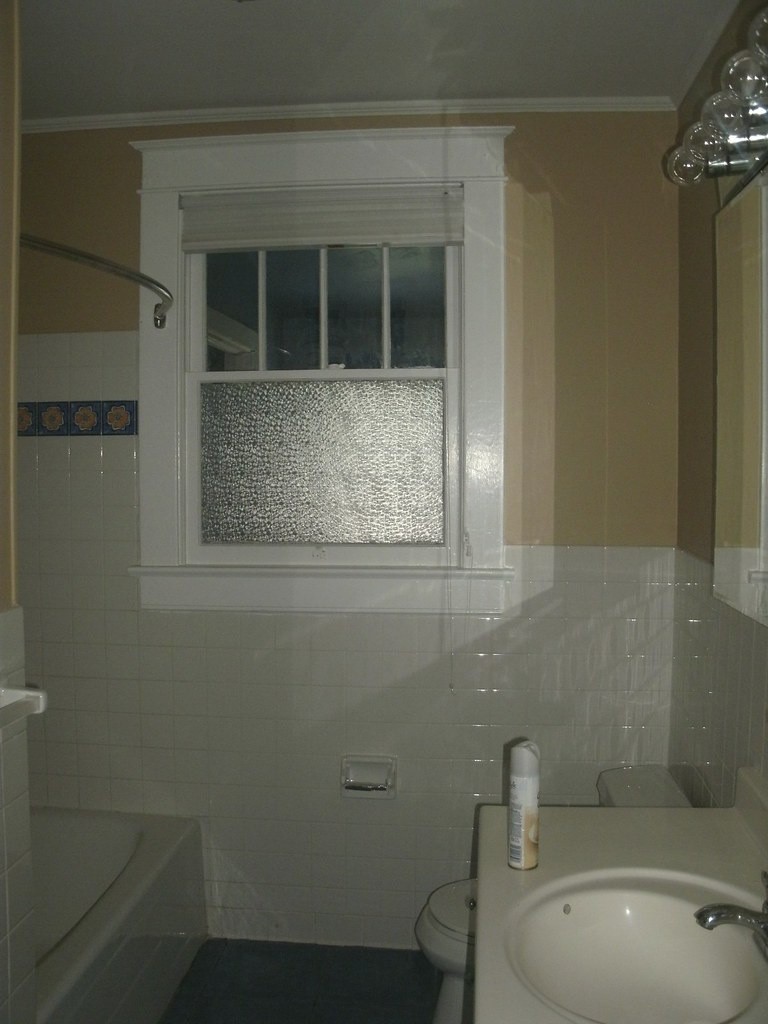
[{"x1": 413, "y1": 762, "x2": 698, "y2": 1024}]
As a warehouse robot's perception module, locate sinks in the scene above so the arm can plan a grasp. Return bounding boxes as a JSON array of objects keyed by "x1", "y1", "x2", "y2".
[{"x1": 496, "y1": 863, "x2": 768, "y2": 1024}]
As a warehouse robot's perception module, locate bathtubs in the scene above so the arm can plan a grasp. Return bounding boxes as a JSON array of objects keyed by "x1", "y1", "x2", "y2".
[{"x1": 34, "y1": 804, "x2": 210, "y2": 1024}]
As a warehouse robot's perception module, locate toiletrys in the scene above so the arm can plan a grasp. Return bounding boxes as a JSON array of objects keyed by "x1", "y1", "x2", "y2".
[{"x1": 505, "y1": 739, "x2": 541, "y2": 872}]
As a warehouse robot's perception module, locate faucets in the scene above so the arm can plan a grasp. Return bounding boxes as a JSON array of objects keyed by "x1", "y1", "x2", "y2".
[{"x1": 691, "y1": 868, "x2": 768, "y2": 963}]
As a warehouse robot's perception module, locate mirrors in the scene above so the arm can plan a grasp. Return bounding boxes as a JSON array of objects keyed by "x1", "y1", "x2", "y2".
[{"x1": 713, "y1": 164, "x2": 768, "y2": 628}]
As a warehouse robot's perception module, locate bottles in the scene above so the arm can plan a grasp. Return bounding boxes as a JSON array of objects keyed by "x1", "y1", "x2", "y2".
[{"x1": 507, "y1": 739, "x2": 542, "y2": 870}]
